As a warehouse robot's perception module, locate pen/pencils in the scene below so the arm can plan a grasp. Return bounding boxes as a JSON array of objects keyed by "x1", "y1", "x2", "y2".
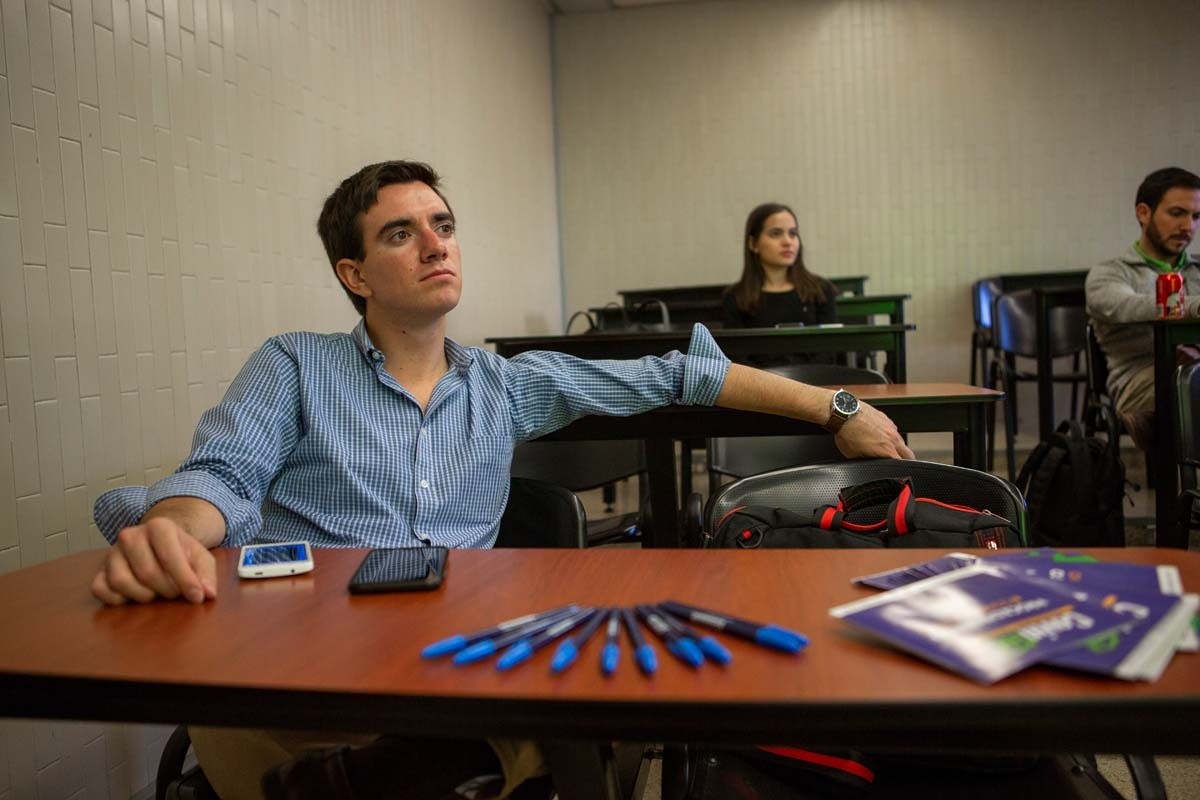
[
  {"x1": 658, "y1": 602, "x2": 800, "y2": 651},
  {"x1": 601, "y1": 608, "x2": 621, "y2": 672},
  {"x1": 421, "y1": 605, "x2": 576, "y2": 657},
  {"x1": 634, "y1": 605, "x2": 705, "y2": 666},
  {"x1": 550, "y1": 607, "x2": 610, "y2": 672},
  {"x1": 495, "y1": 607, "x2": 598, "y2": 670},
  {"x1": 623, "y1": 609, "x2": 657, "y2": 673},
  {"x1": 643, "y1": 604, "x2": 732, "y2": 663},
  {"x1": 453, "y1": 607, "x2": 579, "y2": 664},
  {"x1": 669, "y1": 600, "x2": 808, "y2": 645}
]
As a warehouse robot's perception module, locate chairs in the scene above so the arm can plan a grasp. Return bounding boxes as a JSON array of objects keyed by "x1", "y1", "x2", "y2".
[
  {"x1": 970, "y1": 269, "x2": 1200, "y2": 551},
  {"x1": 711, "y1": 362, "x2": 909, "y2": 481},
  {"x1": 154, "y1": 476, "x2": 616, "y2": 800},
  {"x1": 703, "y1": 459, "x2": 1169, "y2": 800},
  {"x1": 511, "y1": 439, "x2": 649, "y2": 544}
]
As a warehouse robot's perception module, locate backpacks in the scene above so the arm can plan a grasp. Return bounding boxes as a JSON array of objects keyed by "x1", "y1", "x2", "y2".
[{"x1": 1016, "y1": 419, "x2": 1141, "y2": 550}]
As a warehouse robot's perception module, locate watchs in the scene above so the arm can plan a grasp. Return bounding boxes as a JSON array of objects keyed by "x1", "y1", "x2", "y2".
[{"x1": 824, "y1": 389, "x2": 860, "y2": 434}]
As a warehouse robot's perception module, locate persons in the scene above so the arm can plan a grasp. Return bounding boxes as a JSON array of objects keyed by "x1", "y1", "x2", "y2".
[
  {"x1": 718, "y1": 203, "x2": 839, "y2": 364},
  {"x1": 92, "y1": 160, "x2": 914, "y2": 800},
  {"x1": 1084, "y1": 165, "x2": 1200, "y2": 453}
]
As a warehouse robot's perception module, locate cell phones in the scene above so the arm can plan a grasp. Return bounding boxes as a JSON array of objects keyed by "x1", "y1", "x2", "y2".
[
  {"x1": 348, "y1": 546, "x2": 449, "y2": 594},
  {"x1": 238, "y1": 541, "x2": 314, "y2": 578}
]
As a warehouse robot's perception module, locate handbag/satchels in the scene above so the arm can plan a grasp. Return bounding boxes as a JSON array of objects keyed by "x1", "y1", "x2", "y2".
[
  {"x1": 563, "y1": 300, "x2": 675, "y2": 335},
  {"x1": 707, "y1": 475, "x2": 1024, "y2": 552}
]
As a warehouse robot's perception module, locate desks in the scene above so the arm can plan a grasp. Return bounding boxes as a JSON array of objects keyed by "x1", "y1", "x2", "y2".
[
  {"x1": 1, "y1": 548, "x2": 1199, "y2": 800},
  {"x1": 484, "y1": 275, "x2": 1004, "y2": 548}
]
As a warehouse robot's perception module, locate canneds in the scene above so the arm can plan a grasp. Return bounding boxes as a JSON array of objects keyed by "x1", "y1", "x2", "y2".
[{"x1": 1156, "y1": 272, "x2": 1185, "y2": 319}]
